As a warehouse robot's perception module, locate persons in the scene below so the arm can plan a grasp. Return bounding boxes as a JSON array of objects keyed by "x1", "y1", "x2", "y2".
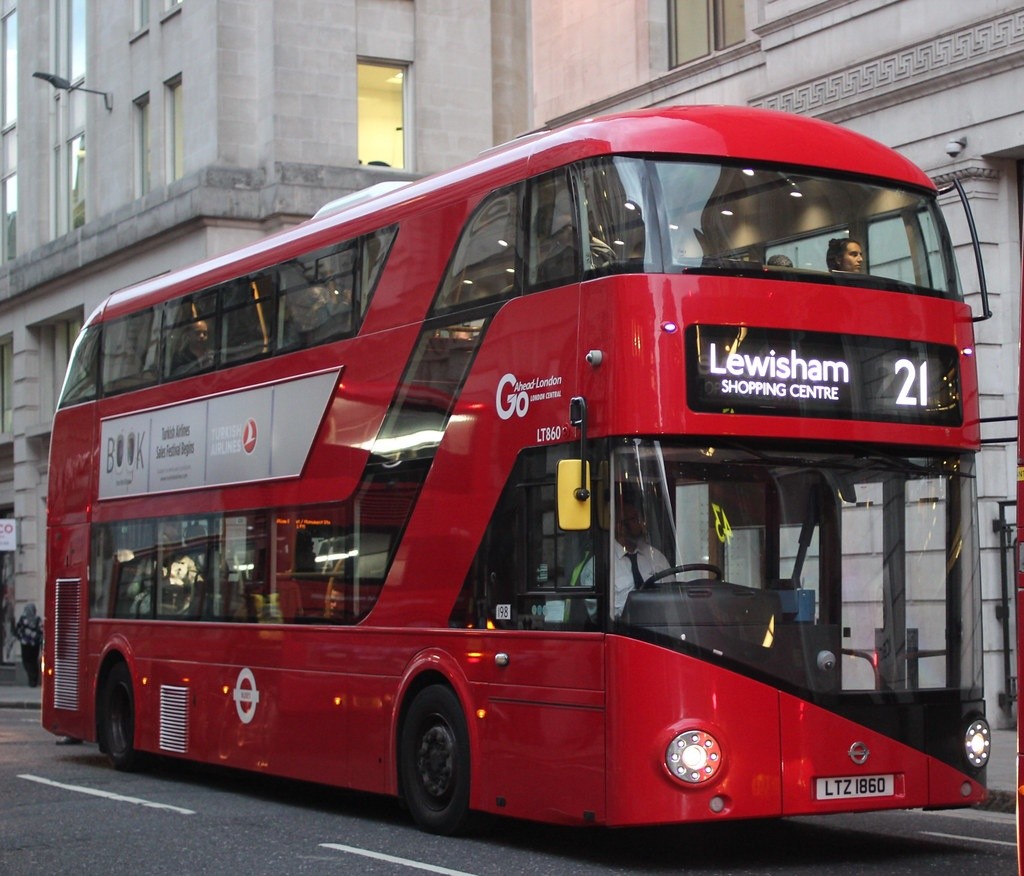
[
  {"x1": 12, "y1": 603, "x2": 44, "y2": 687},
  {"x1": 581, "y1": 481, "x2": 677, "y2": 620},
  {"x1": 306, "y1": 587, "x2": 349, "y2": 621},
  {"x1": 127, "y1": 522, "x2": 208, "y2": 617},
  {"x1": 535, "y1": 214, "x2": 618, "y2": 281},
  {"x1": 170, "y1": 319, "x2": 213, "y2": 369},
  {"x1": 826, "y1": 238, "x2": 863, "y2": 274}
]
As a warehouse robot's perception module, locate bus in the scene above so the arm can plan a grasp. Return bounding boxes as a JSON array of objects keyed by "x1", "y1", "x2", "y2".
[{"x1": 41, "y1": 106, "x2": 991, "y2": 835}]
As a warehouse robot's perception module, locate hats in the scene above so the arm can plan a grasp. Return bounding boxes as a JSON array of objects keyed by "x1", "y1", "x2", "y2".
[{"x1": 155, "y1": 525, "x2": 181, "y2": 553}]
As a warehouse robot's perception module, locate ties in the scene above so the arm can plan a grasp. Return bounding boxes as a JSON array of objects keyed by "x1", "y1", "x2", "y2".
[{"x1": 625, "y1": 552, "x2": 643, "y2": 589}]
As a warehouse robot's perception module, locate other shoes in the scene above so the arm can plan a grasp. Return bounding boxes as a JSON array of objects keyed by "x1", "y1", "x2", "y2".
[{"x1": 55, "y1": 734, "x2": 83, "y2": 747}]
